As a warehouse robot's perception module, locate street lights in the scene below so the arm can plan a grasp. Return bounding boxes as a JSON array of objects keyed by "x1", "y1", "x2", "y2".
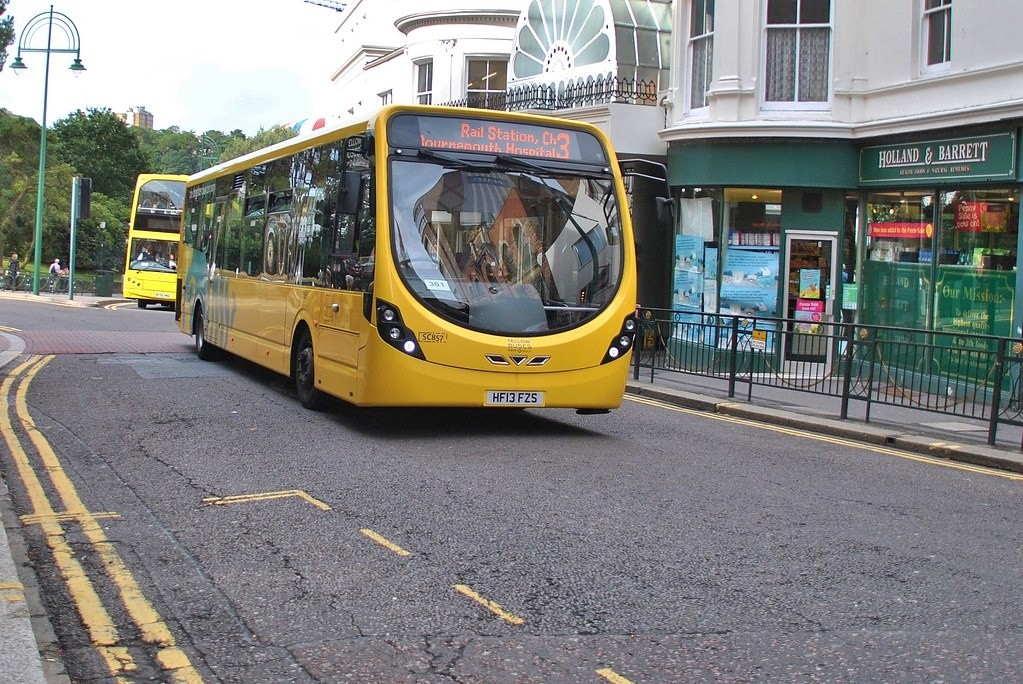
[{"x1": 10, "y1": 3, "x2": 84, "y2": 294}]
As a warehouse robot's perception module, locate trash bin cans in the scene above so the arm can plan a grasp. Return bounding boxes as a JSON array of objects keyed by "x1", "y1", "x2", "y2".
[{"x1": 95, "y1": 270, "x2": 114, "y2": 297}]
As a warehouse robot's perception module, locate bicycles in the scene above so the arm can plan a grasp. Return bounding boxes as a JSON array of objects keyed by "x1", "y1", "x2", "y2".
[{"x1": 1, "y1": 266, "x2": 94, "y2": 296}]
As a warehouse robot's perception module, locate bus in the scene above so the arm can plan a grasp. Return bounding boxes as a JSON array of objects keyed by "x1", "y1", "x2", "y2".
[
  {"x1": 124, "y1": 173, "x2": 194, "y2": 308},
  {"x1": 181, "y1": 99, "x2": 643, "y2": 420}
]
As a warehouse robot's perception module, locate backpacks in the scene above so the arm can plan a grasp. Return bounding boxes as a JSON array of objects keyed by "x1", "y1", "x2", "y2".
[{"x1": 50, "y1": 266, "x2": 56, "y2": 275}]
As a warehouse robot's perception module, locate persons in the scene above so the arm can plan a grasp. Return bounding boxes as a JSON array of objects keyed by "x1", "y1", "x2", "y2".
[
  {"x1": 7, "y1": 254, "x2": 20, "y2": 291},
  {"x1": 49, "y1": 258, "x2": 61, "y2": 293},
  {"x1": 136, "y1": 243, "x2": 160, "y2": 263}
]
